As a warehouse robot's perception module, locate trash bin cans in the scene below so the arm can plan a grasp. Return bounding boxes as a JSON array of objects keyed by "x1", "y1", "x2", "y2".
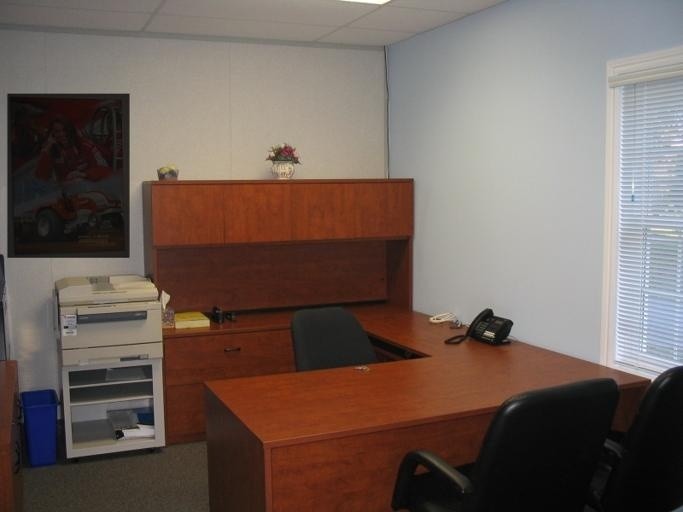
[{"x1": 20, "y1": 389, "x2": 60, "y2": 468}]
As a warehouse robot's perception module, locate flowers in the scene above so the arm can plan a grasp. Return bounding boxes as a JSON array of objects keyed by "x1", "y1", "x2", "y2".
[{"x1": 262, "y1": 143, "x2": 302, "y2": 166}]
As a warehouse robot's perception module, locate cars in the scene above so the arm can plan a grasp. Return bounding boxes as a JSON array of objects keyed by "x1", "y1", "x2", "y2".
[{"x1": 14, "y1": 186, "x2": 122, "y2": 244}]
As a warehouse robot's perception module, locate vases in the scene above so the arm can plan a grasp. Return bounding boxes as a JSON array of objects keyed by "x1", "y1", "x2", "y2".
[{"x1": 271, "y1": 161, "x2": 295, "y2": 180}]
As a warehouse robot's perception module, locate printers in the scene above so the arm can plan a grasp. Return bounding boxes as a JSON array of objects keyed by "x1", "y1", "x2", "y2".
[{"x1": 56, "y1": 274, "x2": 163, "y2": 365}]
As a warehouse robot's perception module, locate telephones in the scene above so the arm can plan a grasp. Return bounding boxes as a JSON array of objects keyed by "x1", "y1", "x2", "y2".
[{"x1": 466, "y1": 309, "x2": 513, "y2": 344}]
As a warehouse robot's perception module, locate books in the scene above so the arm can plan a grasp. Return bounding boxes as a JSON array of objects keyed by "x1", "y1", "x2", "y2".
[{"x1": 173, "y1": 310, "x2": 210, "y2": 329}]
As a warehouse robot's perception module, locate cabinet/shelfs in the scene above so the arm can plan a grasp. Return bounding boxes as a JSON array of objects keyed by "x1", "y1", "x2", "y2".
[
  {"x1": 57, "y1": 357, "x2": 165, "y2": 465},
  {"x1": 0, "y1": 360, "x2": 26, "y2": 512}
]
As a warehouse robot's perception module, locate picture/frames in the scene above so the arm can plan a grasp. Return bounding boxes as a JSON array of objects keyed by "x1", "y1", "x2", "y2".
[{"x1": 6, "y1": 93, "x2": 131, "y2": 260}]
